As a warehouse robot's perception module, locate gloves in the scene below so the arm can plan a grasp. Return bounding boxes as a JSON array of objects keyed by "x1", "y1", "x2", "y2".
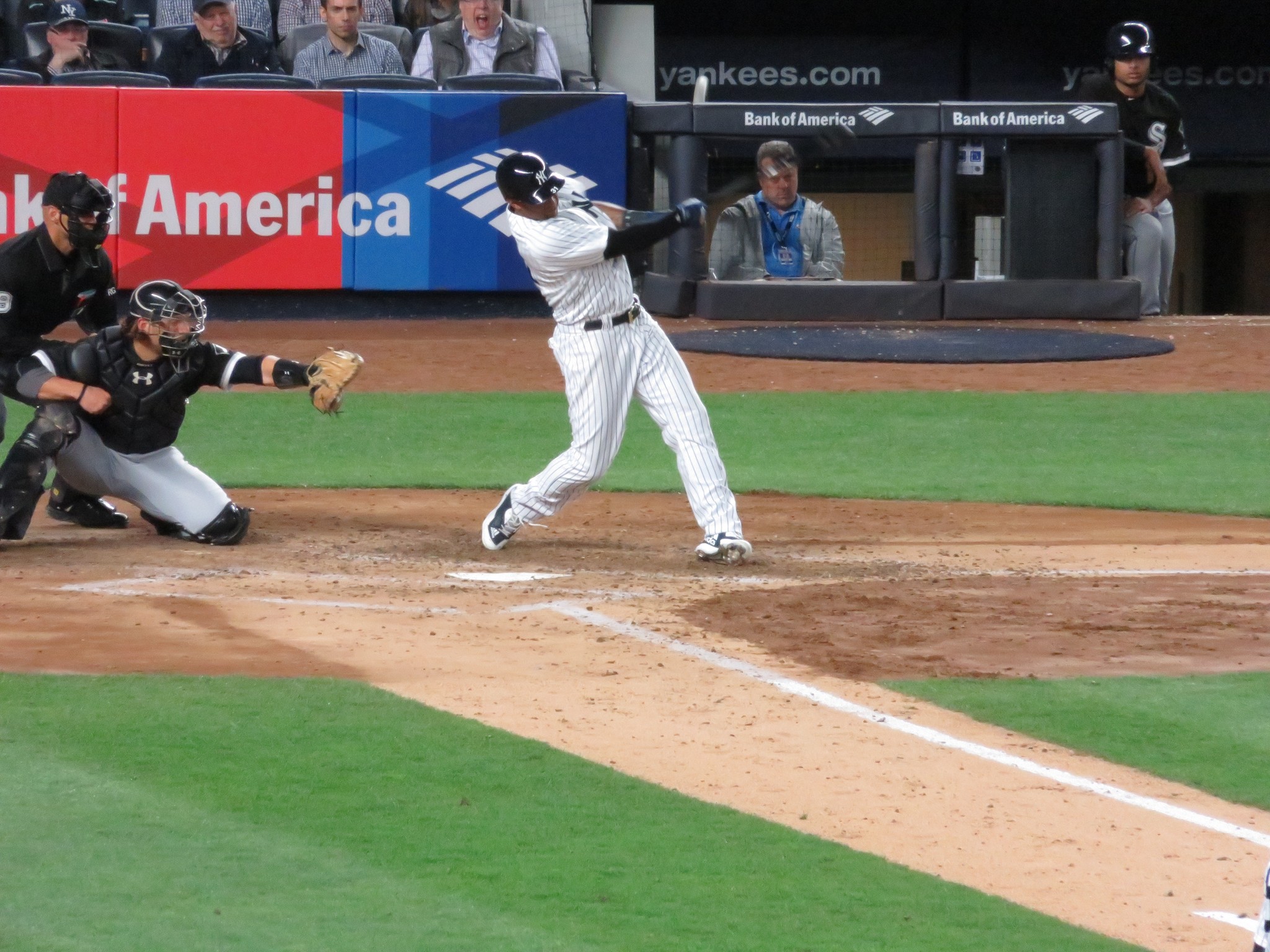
[{"x1": 677, "y1": 198, "x2": 710, "y2": 227}]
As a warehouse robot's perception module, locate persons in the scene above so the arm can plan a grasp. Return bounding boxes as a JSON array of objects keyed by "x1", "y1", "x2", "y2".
[
  {"x1": 155, "y1": 0, "x2": 565, "y2": 92},
  {"x1": 1080, "y1": 23, "x2": 1190, "y2": 317},
  {"x1": 0, "y1": 0, "x2": 136, "y2": 86},
  {"x1": 707, "y1": 141, "x2": 845, "y2": 283},
  {"x1": 482, "y1": 151, "x2": 752, "y2": 567}
]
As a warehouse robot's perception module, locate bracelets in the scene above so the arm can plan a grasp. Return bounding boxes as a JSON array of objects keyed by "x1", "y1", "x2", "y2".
[
  {"x1": 0, "y1": 171, "x2": 364, "y2": 546},
  {"x1": 76, "y1": 384, "x2": 87, "y2": 403}
]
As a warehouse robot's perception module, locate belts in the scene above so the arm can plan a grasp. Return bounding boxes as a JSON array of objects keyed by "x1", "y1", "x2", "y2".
[{"x1": 585, "y1": 300, "x2": 641, "y2": 331}]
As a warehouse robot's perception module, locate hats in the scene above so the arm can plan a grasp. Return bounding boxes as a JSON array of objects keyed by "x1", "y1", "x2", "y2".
[
  {"x1": 42, "y1": 172, "x2": 95, "y2": 213},
  {"x1": 193, "y1": 0, "x2": 235, "y2": 14},
  {"x1": 47, "y1": 0, "x2": 89, "y2": 27}
]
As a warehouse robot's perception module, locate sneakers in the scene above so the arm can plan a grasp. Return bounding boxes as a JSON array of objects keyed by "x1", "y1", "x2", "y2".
[
  {"x1": 140, "y1": 510, "x2": 174, "y2": 536},
  {"x1": 694, "y1": 531, "x2": 753, "y2": 567},
  {"x1": 47, "y1": 494, "x2": 130, "y2": 527},
  {"x1": 481, "y1": 485, "x2": 548, "y2": 550}
]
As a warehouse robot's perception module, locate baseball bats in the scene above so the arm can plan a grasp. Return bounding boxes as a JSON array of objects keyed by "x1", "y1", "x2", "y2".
[{"x1": 701, "y1": 126, "x2": 857, "y2": 204}]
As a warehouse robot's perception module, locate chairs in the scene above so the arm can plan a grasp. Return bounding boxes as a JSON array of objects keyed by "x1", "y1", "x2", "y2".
[
  {"x1": 0, "y1": 68, "x2": 44, "y2": 86},
  {"x1": 15, "y1": 21, "x2": 142, "y2": 72},
  {"x1": 195, "y1": 72, "x2": 316, "y2": 89},
  {"x1": 317, "y1": 73, "x2": 439, "y2": 91},
  {"x1": 47, "y1": 70, "x2": 172, "y2": 89},
  {"x1": 146, "y1": 23, "x2": 266, "y2": 74},
  {"x1": 278, "y1": 22, "x2": 412, "y2": 75},
  {"x1": 442, "y1": 73, "x2": 562, "y2": 91}
]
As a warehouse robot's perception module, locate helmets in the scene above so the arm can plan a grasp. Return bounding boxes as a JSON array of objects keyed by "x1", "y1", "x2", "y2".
[
  {"x1": 129, "y1": 279, "x2": 189, "y2": 322},
  {"x1": 1107, "y1": 21, "x2": 1153, "y2": 56},
  {"x1": 496, "y1": 152, "x2": 564, "y2": 204}
]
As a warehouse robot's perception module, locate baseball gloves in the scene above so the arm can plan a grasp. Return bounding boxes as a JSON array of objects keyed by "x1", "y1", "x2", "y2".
[{"x1": 306, "y1": 347, "x2": 366, "y2": 415}]
